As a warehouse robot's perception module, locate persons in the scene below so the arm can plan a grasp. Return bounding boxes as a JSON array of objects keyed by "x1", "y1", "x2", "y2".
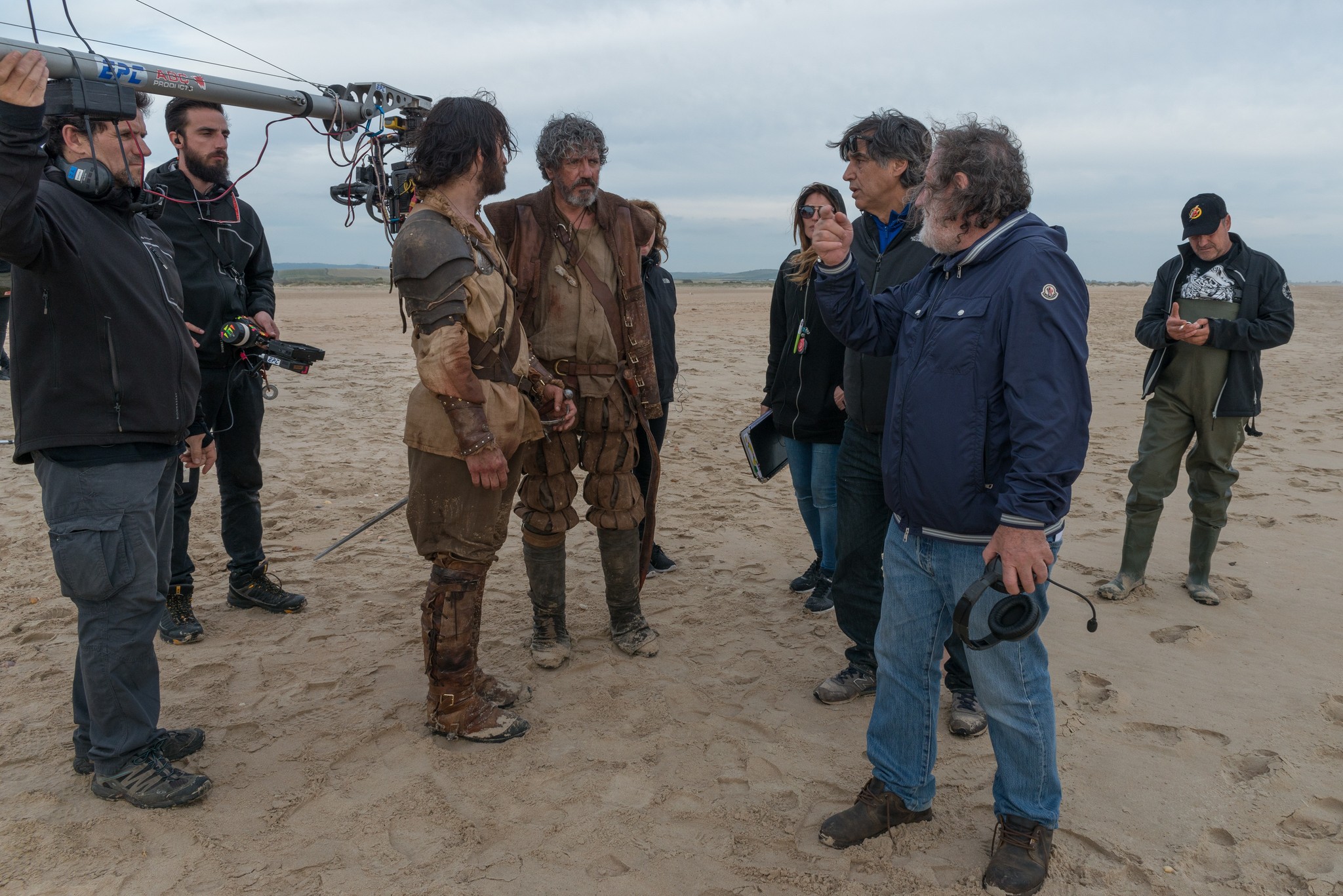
[
  {"x1": 0, "y1": 48, "x2": 214, "y2": 807},
  {"x1": 760, "y1": 182, "x2": 847, "y2": 614},
  {"x1": 630, "y1": 201, "x2": 678, "y2": 579},
  {"x1": 144, "y1": 98, "x2": 307, "y2": 646},
  {"x1": 394, "y1": 91, "x2": 576, "y2": 744},
  {"x1": 483, "y1": 114, "x2": 662, "y2": 670},
  {"x1": 1097, "y1": 194, "x2": 1295, "y2": 605},
  {"x1": 812, "y1": 115, "x2": 1091, "y2": 896},
  {"x1": 813, "y1": 109, "x2": 989, "y2": 738}
]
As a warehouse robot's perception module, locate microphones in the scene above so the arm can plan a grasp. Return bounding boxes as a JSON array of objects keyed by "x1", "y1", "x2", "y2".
[{"x1": 1045, "y1": 577, "x2": 1098, "y2": 633}]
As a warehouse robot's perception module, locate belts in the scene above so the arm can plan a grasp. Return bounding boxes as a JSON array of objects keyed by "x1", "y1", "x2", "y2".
[
  {"x1": 473, "y1": 366, "x2": 533, "y2": 397},
  {"x1": 540, "y1": 358, "x2": 618, "y2": 377}
]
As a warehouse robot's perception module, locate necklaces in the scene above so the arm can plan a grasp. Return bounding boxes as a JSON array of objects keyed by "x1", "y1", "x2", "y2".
[
  {"x1": 553, "y1": 201, "x2": 595, "y2": 293},
  {"x1": 436, "y1": 187, "x2": 469, "y2": 223}
]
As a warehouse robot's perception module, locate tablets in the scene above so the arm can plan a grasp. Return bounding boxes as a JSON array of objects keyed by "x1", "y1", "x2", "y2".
[{"x1": 739, "y1": 408, "x2": 789, "y2": 484}]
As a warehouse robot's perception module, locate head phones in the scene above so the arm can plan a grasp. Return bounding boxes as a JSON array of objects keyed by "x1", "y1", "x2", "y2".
[
  {"x1": 953, "y1": 554, "x2": 1042, "y2": 650},
  {"x1": 52, "y1": 151, "x2": 152, "y2": 215}
]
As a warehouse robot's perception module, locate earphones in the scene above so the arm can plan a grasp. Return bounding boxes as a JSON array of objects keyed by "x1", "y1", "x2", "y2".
[{"x1": 174, "y1": 129, "x2": 181, "y2": 145}]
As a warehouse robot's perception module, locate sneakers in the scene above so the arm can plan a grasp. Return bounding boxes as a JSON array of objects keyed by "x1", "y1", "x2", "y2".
[
  {"x1": 813, "y1": 666, "x2": 876, "y2": 705},
  {"x1": 803, "y1": 572, "x2": 835, "y2": 614},
  {"x1": 158, "y1": 583, "x2": 204, "y2": 644},
  {"x1": 73, "y1": 727, "x2": 205, "y2": 775},
  {"x1": 649, "y1": 541, "x2": 677, "y2": 572},
  {"x1": 789, "y1": 557, "x2": 823, "y2": 594},
  {"x1": 645, "y1": 563, "x2": 657, "y2": 578},
  {"x1": 949, "y1": 691, "x2": 989, "y2": 737},
  {"x1": 982, "y1": 815, "x2": 1054, "y2": 896},
  {"x1": 91, "y1": 737, "x2": 211, "y2": 808},
  {"x1": 226, "y1": 560, "x2": 307, "y2": 614},
  {"x1": 818, "y1": 776, "x2": 933, "y2": 849}
]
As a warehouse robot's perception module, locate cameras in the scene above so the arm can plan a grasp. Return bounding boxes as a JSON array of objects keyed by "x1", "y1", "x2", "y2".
[{"x1": 218, "y1": 316, "x2": 325, "y2": 374}]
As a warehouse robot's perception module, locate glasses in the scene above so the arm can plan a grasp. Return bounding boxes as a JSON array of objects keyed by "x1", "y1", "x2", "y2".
[
  {"x1": 798, "y1": 203, "x2": 836, "y2": 219},
  {"x1": 842, "y1": 134, "x2": 874, "y2": 159}
]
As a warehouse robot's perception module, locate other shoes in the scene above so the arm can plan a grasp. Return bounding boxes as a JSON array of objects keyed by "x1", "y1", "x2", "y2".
[
  {"x1": 634, "y1": 637, "x2": 659, "y2": 657},
  {"x1": 430, "y1": 705, "x2": 531, "y2": 743},
  {"x1": 498, "y1": 685, "x2": 533, "y2": 709},
  {"x1": 531, "y1": 649, "x2": 565, "y2": 669}
]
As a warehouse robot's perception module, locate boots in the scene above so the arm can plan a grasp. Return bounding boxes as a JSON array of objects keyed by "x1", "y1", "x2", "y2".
[
  {"x1": 1097, "y1": 523, "x2": 1156, "y2": 600},
  {"x1": 1186, "y1": 522, "x2": 1223, "y2": 607}
]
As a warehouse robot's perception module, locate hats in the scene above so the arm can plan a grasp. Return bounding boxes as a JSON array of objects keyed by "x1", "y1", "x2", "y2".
[{"x1": 1181, "y1": 192, "x2": 1227, "y2": 242}]
